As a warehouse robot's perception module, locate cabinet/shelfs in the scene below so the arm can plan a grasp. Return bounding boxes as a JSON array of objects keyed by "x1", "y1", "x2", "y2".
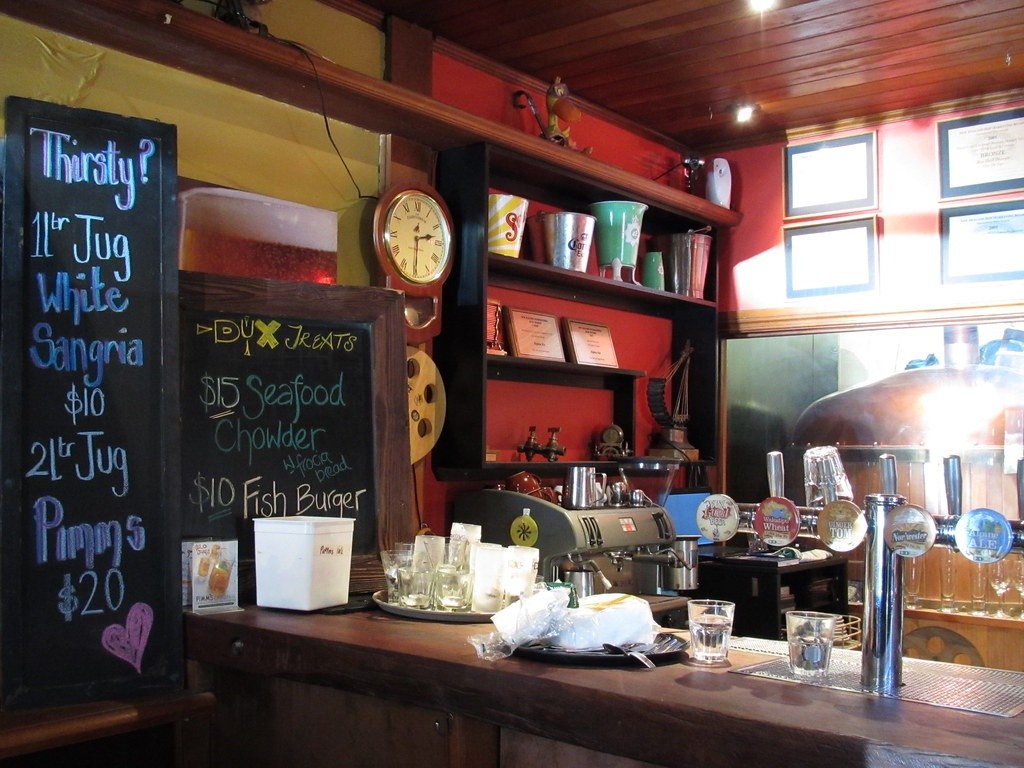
[{"x1": 432, "y1": 140, "x2": 725, "y2": 495}]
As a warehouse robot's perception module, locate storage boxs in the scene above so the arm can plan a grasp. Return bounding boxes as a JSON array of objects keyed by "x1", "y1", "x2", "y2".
[{"x1": 253, "y1": 517, "x2": 356, "y2": 611}]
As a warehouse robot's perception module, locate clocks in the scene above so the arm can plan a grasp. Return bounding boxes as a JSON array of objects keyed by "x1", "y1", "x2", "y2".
[{"x1": 372, "y1": 181, "x2": 455, "y2": 345}]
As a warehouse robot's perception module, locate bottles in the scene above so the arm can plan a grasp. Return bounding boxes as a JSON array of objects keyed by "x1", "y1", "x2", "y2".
[{"x1": 642, "y1": 251, "x2": 664, "y2": 292}]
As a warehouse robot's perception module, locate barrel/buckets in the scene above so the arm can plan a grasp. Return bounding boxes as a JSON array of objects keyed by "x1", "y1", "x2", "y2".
[
  {"x1": 488, "y1": 193, "x2": 530, "y2": 258},
  {"x1": 653, "y1": 225, "x2": 712, "y2": 299},
  {"x1": 526, "y1": 211, "x2": 597, "y2": 274},
  {"x1": 588, "y1": 201, "x2": 649, "y2": 286}
]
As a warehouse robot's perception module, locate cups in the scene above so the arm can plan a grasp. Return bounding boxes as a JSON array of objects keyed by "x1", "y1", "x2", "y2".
[
  {"x1": 493, "y1": 466, "x2": 653, "y2": 507},
  {"x1": 199, "y1": 548, "x2": 235, "y2": 595},
  {"x1": 380, "y1": 523, "x2": 539, "y2": 613},
  {"x1": 687, "y1": 599, "x2": 734, "y2": 662},
  {"x1": 803, "y1": 446, "x2": 854, "y2": 510},
  {"x1": 786, "y1": 611, "x2": 836, "y2": 675}
]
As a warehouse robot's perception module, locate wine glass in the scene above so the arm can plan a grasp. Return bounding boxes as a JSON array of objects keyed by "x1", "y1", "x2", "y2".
[{"x1": 991, "y1": 554, "x2": 1024, "y2": 620}]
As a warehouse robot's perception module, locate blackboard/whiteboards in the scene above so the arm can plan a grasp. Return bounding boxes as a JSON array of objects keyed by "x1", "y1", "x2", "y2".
[
  {"x1": 0, "y1": 97, "x2": 179, "y2": 753},
  {"x1": 178, "y1": 270, "x2": 416, "y2": 599}
]
